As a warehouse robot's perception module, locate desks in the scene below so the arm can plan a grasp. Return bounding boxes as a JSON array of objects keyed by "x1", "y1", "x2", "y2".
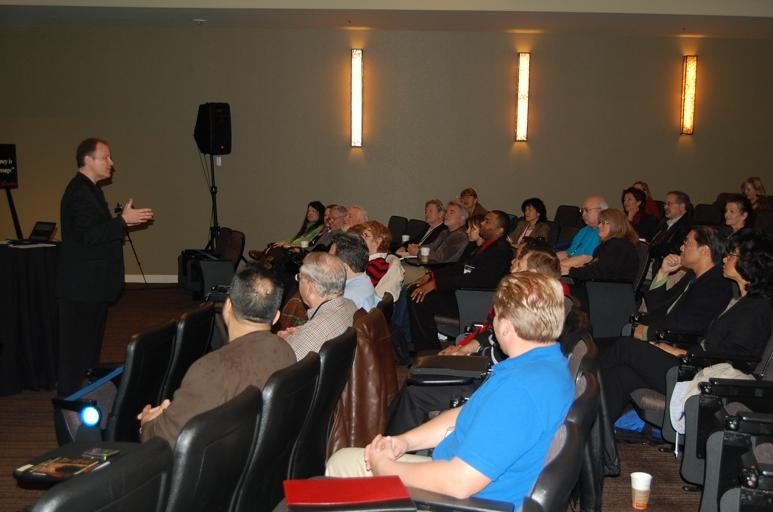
[{"x1": 0, "y1": 237, "x2": 63, "y2": 397}]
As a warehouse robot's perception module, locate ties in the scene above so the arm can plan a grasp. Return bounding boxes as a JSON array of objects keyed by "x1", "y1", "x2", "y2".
[{"x1": 656, "y1": 223, "x2": 668, "y2": 245}]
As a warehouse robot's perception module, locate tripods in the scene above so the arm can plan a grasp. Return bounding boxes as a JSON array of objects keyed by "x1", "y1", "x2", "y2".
[
  {"x1": 125, "y1": 230, "x2": 147, "y2": 285},
  {"x1": 204, "y1": 155, "x2": 247, "y2": 264}
]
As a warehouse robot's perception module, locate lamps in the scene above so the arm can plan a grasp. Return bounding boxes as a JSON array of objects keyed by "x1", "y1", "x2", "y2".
[
  {"x1": 346, "y1": 48, "x2": 364, "y2": 150},
  {"x1": 677, "y1": 54, "x2": 699, "y2": 136},
  {"x1": 513, "y1": 50, "x2": 531, "y2": 144}
]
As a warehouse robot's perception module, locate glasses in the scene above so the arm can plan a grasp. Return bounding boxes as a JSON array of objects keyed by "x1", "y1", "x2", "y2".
[
  {"x1": 600, "y1": 220, "x2": 608, "y2": 225},
  {"x1": 726, "y1": 251, "x2": 739, "y2": 259},
  {"x1": 295, "y1": 274, "x2": 306, "y2": 283},
  {"x1": 579, "y1": 207, "x2": 598, "y2": 214}
]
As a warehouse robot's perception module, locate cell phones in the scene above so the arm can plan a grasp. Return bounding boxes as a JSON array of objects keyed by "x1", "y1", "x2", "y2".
[{"x1": 83, "y1": 447, "x2": 120, "y2": 458}]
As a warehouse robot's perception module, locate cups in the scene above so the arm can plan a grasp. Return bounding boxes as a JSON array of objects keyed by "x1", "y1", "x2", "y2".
[
  {"x1": 401, "y1": 234, "x2": 409, "y2": 247},
  {"x1": 628, "y1": 471, "x2": 652, "y2": 510},
  {"x1": 420, "y1": 247, "x2": 430, "y2": 265},
  {"x1": 300, "y1": 241, "x2": 307, "y2": 254}
]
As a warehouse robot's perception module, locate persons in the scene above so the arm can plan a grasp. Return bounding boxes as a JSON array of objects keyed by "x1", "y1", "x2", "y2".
[{"x1": 59, "y1": 138, "x2": 155, "y2": 397}]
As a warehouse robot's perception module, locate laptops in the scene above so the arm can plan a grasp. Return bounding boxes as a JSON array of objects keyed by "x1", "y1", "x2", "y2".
[{"x1": 8, "y1": 221, "x2": 56, "y2": 245}]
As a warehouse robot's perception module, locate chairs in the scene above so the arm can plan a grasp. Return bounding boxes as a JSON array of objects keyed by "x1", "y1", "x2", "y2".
[
  {"x1": 621, "y1": 311, "x2": 772, "y2": 512},
  {"x1": 387, "y1": 216, "x2": 431, "y2": 258},
  {"x1": 550, "y1": 205, "x2": 582, "y2": 243},
  {"x1": 507, "y1": 213, "x2": 519, "y2": 233},
  {"x1": 575, "y1": 240, "x2": 651, "y2": 339},
  {"x1": 27, "y1": 283, "x2": 395, "y2": 512},
  {"x1": 176, "y1": 226, "x2": 246, "y2": 301},
  {"x1": 271, "y1": 277, "x2": 620, "y2": 512}
]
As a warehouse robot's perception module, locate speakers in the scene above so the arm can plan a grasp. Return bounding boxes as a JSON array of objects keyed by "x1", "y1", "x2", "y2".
[{"x1": 194, "y1": 103, "x2": 232, "y2": 154}]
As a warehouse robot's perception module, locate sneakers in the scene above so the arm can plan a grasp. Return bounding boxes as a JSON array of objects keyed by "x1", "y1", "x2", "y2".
[{"x1": 249, "y1": 250, "x2": 262, "y2": 259}]
